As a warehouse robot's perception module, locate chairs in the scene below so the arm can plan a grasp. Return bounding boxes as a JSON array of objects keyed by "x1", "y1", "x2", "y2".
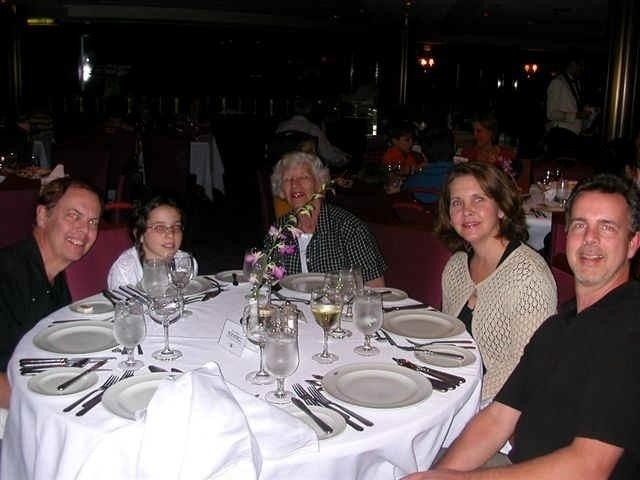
[{"x1": 1, "y1": 141, "x2": 640, "y2": 317}]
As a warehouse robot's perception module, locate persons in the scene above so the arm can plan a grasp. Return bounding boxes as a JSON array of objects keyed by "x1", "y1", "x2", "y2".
[
  {"x1": 2, "y1": 99, "x2": 56, "y2": 162},
  {"x1": 263, "y1": 152, "x2": 389, "y2": 288},
  {"x1": 270, "y1": 96, "x2": 353, "y2": 165},
  {"x1": 106, "y1": 190, "x2": 198, "y2": 290},
  {"x1": 596, "y1": 135, "x2": 640, "y2": 185},
  {"x1": 97, "y1": 94, "x2": 136, "y2": 137},
  {"x1": 402, "y1": 171, "x2": 639, "y2": 479},
  {"x1": 461, "y1": 114, "x2": 519, "y2": 169},
  {"x1": 384, "y1": 121, "x2": 429, "y2": 176},
  {"x1": 432, "y1": 161, "x2": 559, "y2": 412},
  {"x1": 0, "y1": 177, "x2": 102, "y2": 436},
  {"x1": 545, "y1": 56, "x2": 591, "y2": 167}
]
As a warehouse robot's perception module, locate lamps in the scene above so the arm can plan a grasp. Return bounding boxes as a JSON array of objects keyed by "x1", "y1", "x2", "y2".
[
  {"x1": 418, "y1": 58, "x2": 436, "y2": 74},
  {"x1": 523, "y1": 63, "x2": 539, "y2": 81}
]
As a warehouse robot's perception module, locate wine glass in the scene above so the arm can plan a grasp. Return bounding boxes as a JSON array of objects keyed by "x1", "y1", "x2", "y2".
[{"x1": 540, "y1": 167, "x2": 570, "y2": 214}]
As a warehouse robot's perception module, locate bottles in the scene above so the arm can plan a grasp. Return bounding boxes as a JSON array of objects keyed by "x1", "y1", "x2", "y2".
[
  {"x1": 382, "y1": 159, "x2": 401, "y2": 177},
  {"x1": 106, "y1": 175, "x2": 116, "y2": 202}
]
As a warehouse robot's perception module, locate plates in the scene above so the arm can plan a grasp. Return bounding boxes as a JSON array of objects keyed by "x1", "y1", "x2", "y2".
[
  {"x1": 33, "y1": 250, "x2": 477, "y2": 443},
  {"x1": 9, "y1": 163, "x2": 51, "y2": 180}
]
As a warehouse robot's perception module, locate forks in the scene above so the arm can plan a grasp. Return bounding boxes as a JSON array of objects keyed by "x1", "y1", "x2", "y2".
[{"x1": 17, "y1": 353, "x2": 134, "y2": 418}]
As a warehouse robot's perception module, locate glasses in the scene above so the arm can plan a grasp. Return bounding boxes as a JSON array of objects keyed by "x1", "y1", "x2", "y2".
[{"x1": 146, "y1": 224, "x2": 182, "y2": 232}]
